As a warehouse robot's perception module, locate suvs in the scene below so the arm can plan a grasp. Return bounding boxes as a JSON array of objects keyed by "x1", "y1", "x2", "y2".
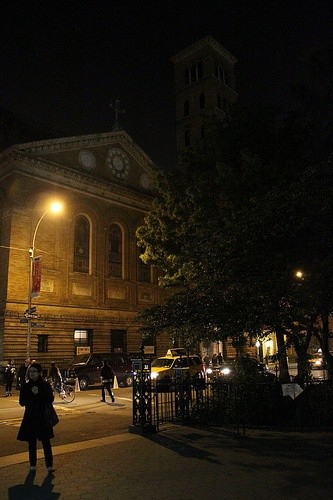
[{"x1": 67, "y1": 352, "x2": 133, "y2": 391}]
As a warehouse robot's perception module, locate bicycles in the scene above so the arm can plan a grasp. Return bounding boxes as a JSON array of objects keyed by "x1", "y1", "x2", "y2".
[{"x1": 44, "y1": 377, "x2": 75, "y2": 403}]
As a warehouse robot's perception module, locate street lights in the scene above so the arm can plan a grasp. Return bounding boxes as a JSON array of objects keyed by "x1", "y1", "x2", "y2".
[{"x1": 24, "y1": 200, "x2": 63, "y2": 369}]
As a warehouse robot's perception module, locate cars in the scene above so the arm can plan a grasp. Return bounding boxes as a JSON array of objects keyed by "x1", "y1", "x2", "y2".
[
  {"x1": 133, "y1": 347, "x2": 204, "y2": 393},
  {"x1": 308, "y1": 348, "x2": 323, "y2": 371},
  {"x1": 206, "y1": 356, "x2": 270, "y2": 384}
]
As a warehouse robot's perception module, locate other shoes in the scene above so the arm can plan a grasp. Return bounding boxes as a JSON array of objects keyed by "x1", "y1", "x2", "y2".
[
  {"x1": 30, "y1": 466, "x2": 35, "y2": 473},
  {"x1": 100, "y1": 399, "x2": 105, "y2": 402},
  {"x1": 111, "y1": 395, "x2": 115, "y2": 402},
  {"x1": 48, "y1": 466, "x2": 54, "y2": 472}
]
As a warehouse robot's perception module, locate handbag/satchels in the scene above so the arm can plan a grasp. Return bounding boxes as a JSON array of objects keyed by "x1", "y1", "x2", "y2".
[{"x1": 35, "y1": 403, "x2": 58, "y2": 432}]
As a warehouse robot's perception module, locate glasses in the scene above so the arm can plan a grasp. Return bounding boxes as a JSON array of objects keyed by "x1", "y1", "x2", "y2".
[{"x1": 28, "y1": 370, "x2": 39, "y2": 373}]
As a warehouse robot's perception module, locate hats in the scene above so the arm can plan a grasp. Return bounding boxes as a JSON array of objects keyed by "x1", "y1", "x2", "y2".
[{"x1": 30, "y1": 363, "x2": 42, "y2": 372}]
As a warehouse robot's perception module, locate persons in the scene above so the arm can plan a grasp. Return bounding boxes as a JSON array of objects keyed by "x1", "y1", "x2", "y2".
[
  {"x1": 16, "y1": 363, "x2": 60, "y2": 472},
  {"x1": 99, "y1": 360, "x2": 115, "y2": 402},
  {"x1": 204, "y1": 353, "x2": 222, "y2": 366},
  {"x1": 3, "y1": 358, "x2": 76, "y2": 397}
]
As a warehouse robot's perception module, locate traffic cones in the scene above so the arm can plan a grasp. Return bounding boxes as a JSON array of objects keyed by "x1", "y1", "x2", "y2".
[
  {"x1": 111, "y1": 374, "x2": 121, "y2": 390},
  {"x1": 73, "y1": 376, "x2": 82, "y2": 393}
]
